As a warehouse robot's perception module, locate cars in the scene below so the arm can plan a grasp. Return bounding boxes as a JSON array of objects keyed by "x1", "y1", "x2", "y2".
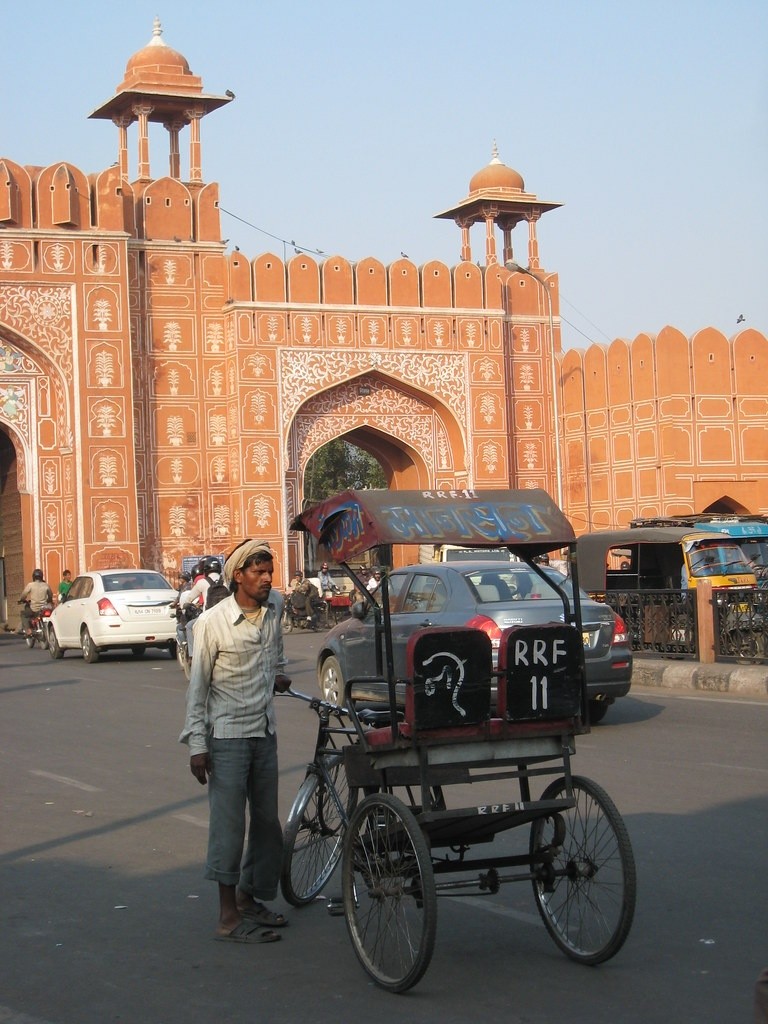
[
  {"x1": 46, "y1": 569, "x2": 180, "y2": 664},
  {"x1": 317, "y1": 559, "x2": 634, "y2": 723}
]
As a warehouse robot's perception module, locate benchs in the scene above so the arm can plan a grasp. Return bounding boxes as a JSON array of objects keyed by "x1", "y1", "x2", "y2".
[{"x1": 606, "y1": 568, "x2": 666, "y2": 604}]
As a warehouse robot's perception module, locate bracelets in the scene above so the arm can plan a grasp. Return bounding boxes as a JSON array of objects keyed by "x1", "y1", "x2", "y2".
[
  {"x1": 194, "y1": 765, "x2": 199, "y2": 769},
  {"x1": 192, "y1": 767, "x2": 195, "y2": 771}
]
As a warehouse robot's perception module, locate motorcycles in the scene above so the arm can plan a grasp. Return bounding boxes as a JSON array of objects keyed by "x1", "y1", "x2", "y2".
[
  {"x1": 170, "y1": 585, "x2": 356, "y2": 683},
  {"x1": 565, "y1": 511, "x2": 768, "y2": 667},
  {"x1": 17, "y1": 600, "x2": 49, "y2": 651}
]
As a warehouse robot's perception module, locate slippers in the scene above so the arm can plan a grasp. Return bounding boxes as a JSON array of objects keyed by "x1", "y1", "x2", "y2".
[
  {"x1": 240, "y1": 902, "x2": 288, "y2": 926},
  {"x1": 215, "y1": 924, "x2": 282, "y2": 944}
]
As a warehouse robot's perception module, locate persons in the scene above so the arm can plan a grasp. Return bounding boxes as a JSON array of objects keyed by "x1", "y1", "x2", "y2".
[
  {"x1": 177, "y1": 536, "x2": 292, "y2": 945},
  {"x1": 56, "y1": 569, "x2": 74, "y2": 607},
  {"x1": 480, "y1": 551, "x2": 550, "y2": 599},
  {"x1": 17, "y1": 569, "x2": 54, "y2": 640},
  {"x1": 170, "y1": 554, "x2": 232, "y2": 666},
  {"x1": 284, "y1": 560, "x2": 383, "y2": 629}
]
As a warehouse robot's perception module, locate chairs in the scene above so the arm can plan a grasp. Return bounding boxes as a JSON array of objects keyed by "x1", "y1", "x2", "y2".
[
  {"x1": 489, "y1": 620, "x2": 580, "y2": 740},
  {"x1": 414, "y1": 584, "x2": 445, "y2": 612},
  {"x1": 143, "y1": 579, "x2": 161, "y2": 589},
  {"x1": 363, "y1": 624, "x2": 493, "y2": 750},
  {"x1": 669, "y1": 574, "x2": 682, "y2": 603},
  {"x1": 475, "y1": 584, "x2": 500, "y2": 601},
  {"x1": 108, "y1": 583, "x2": 123, "y2": 590},
  {"x1": 531, "y1": 581, "x2": 560, "y2": 598}
]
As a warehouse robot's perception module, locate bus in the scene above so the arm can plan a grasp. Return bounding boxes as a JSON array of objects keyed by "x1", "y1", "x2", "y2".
[{"x1": 431, "y1": 543, "x2": 565, "y2": 600}]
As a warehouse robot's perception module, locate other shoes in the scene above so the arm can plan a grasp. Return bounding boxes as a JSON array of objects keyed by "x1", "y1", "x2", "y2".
[{"x1": 27, "y1": 628, "x2": 33, "y2": 636}]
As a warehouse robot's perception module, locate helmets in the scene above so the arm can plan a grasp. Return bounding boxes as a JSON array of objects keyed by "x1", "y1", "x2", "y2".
[
  {"x1": 200, "y1": 554, "x2": 211, "y2": 564},
  {"x1": 33, "y1": 569, "x2": 43, "y2": 578},
  {"x1": 374, "y1": 569, "x2": 380, "y2": 575},
  {"x1": 200, "y1": 557, "x2": 221, "y2": 573},
  {"x1": 180, "y1": 571, "x2": 191, "y2": 581},
  {"x1": 294, "y1": 571, "x2": 303, "y2": 576},
  {"x1": 360, "y1": 565, "x2": 366, "y2": 570},
  {"x1": 321, "y1": 563, "x2": 328, "y2": 569},
  {"x1": 192, "y1": 565, "x2": 203, "y2": 581}
]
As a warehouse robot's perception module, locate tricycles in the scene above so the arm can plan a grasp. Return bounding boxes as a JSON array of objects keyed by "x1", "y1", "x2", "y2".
[{"x1": 274, "y1": 486, "x2": 638, "y2": 995}]
